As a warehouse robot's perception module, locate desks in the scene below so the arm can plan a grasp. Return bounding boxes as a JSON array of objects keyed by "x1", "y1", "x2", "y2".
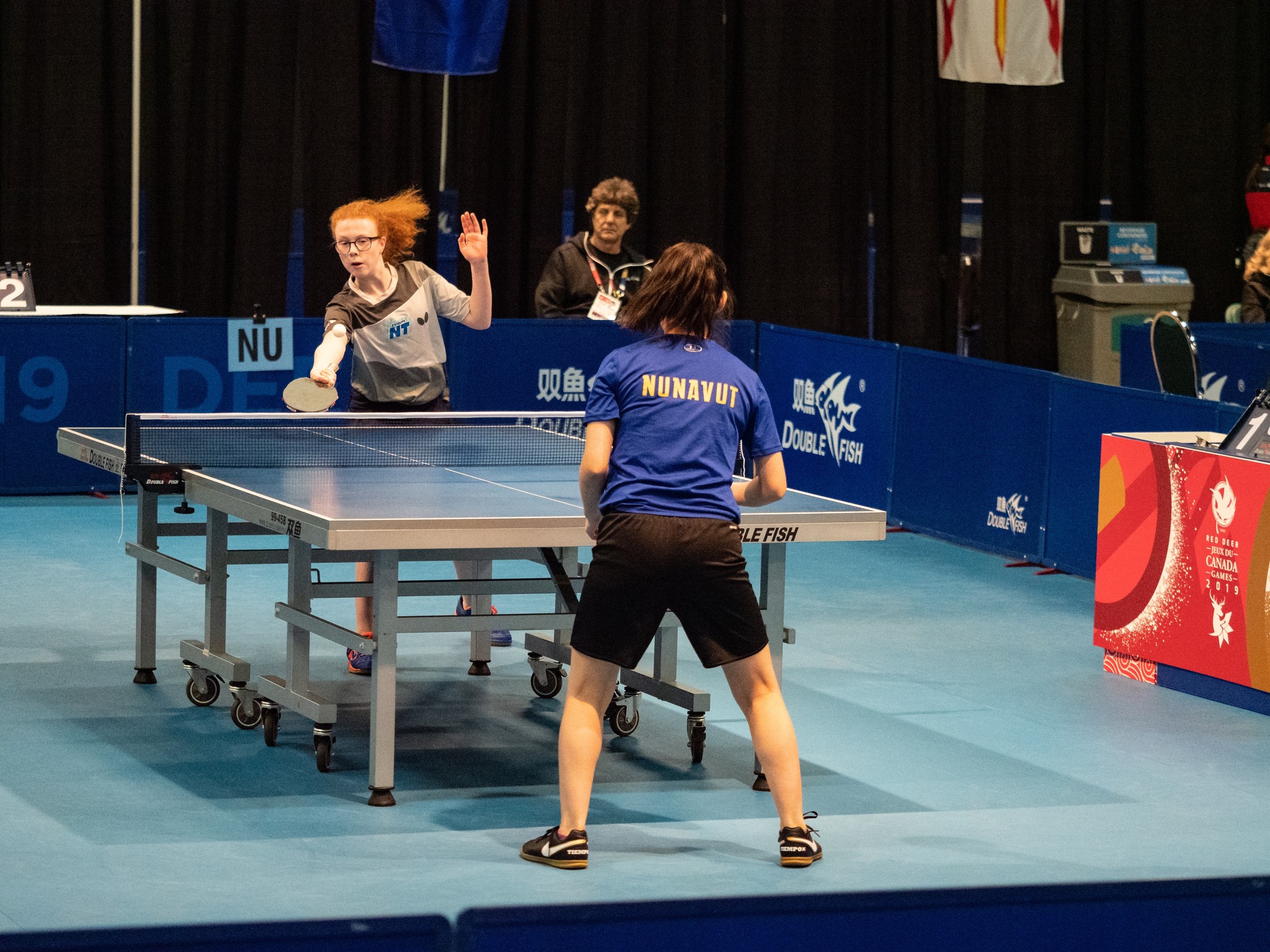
[
  {"x1": 57, "y1": 411, "x2": 888, "y2": 807},
  {"x1": 1097, "y1": 427, "x2": 1270, "y2": 716},
  {"x1": 0, "y1": 305, "x2": 184, "y2": 316}
]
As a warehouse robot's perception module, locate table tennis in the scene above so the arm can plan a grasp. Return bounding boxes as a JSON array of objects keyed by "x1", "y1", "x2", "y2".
[{"x1": 332, "y1": 324, "x2": 346, "y2": 338}]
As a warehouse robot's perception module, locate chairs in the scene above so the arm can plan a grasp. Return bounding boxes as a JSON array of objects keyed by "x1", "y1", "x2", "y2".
[{"x1": 1151, "y1": 311, "x2": 1198, "y2": 399}]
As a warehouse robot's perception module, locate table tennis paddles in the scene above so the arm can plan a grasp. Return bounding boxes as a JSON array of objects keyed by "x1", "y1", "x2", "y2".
[{"x1": 282, "y1": 362, "x2": 339, "y2": 413}]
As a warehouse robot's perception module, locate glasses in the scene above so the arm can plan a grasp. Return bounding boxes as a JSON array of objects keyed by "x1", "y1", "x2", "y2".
[{"x1": 332, "y1": 235, "x2": 379, "y2": 255}]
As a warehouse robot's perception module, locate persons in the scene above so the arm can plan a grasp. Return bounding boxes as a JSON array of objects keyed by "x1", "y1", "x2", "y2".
[
  {"x1": 535, "y1": 178, "x2": 653, "y2": 319},
  {"x1": 1239, "y1": 229, "x2": 1270, "y2": 323},
  {"x1": 309, "y1": 187, "x2": 513, "y2": 674},
  {"x1": 522, "y1": 242, "x2": 823, "y2": 867},
  {"x1": 1244, "y1": 123, "x2": 1270, "y2": 232}
]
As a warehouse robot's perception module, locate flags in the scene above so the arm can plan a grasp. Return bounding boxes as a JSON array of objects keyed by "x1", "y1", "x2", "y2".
[
  {"x1": 937, "y1": 0, "x2": 1065, "y2": 86},
  {"x1": 371, "y1": 0, "x2": 509, "y2": 75}
]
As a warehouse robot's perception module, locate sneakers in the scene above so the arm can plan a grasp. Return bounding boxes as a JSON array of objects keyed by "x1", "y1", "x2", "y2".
[
  {"x1": 779, "y1": 810, "x2": 823, "y2": 865},
  {"x1": 520, "y1": 826, "x2": 589, "y2": 868},
  {"x1": 346, "y1": 632, "x2": 372, "y2": 674},
  {"x1": 454, "y1": 596, "x2": 512, "y2": 644}
]
